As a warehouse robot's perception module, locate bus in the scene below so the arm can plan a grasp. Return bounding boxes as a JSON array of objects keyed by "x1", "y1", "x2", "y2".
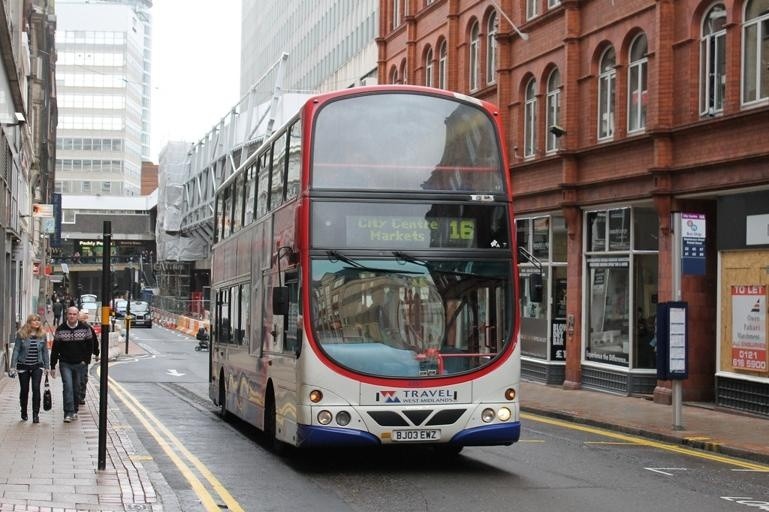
[{"x1": 205, "y1": 82, "x2": 524, "y2": 474}]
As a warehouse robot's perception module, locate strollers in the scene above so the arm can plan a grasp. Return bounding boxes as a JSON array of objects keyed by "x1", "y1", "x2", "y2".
[{"x1": 195, "y1": 327, "x2": 210, "y2": 352}]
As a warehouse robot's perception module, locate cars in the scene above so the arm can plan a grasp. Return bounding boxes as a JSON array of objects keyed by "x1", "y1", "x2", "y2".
[
  {"x1": 80, "y1": 293, "x2": 97, "y2": 313},
  {"x1": 126, "y1": 301, "x2": 152, "y2": 329},
  {"x1": 110, "y1": 298, "x2": 128, "y2": 319}
]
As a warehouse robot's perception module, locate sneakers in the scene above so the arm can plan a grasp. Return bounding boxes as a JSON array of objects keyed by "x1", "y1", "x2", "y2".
[
  {"x1": 79, "y1": 398, "x2": 85, "y2": 404},
  {"x1": 64, "y1": 413, "x2": 78, "y2": 421},
  {"x1": 33, "y1": 415, "x2": 38, "y2": 423},
  {"x1": 21, "y1": 411, "x2": 28, "y2": 419}
]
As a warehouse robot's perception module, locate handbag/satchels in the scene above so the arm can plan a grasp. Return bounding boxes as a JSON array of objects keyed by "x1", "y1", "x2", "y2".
[{"x1": 44, "y1": 389, "x2": 51, "y2": 410}]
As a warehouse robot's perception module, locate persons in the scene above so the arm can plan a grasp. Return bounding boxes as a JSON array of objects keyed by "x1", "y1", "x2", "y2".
[
  {"x1": 7, "y1": 313, "x2": 51, "y2": 424},
  {"x1": 637, "y1": 307, "x2": 650, "y2": 368},
  {"x1": 196, "y1": 305, "x2": 211, "y2": 341},
  {"x1": 76, "y1": 311, "x2": 100, "y2": 405},
  {"x1": 50, "y1": 305, "x2": 95, "y2": 424},
  {"x1": 309, "y1": 271, "x2": 408, "y2": 343},
  {"x1": 50, "y1": 247, "x2": 96, "y2": 264},
  {"x1": 45, "y1": 285, "x2": 84, "y2": 329}
]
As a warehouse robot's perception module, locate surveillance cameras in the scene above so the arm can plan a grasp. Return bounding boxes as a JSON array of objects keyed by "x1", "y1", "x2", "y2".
[{"x1": 13, "y1": 112, "x2": 26, "y2": 126}]
